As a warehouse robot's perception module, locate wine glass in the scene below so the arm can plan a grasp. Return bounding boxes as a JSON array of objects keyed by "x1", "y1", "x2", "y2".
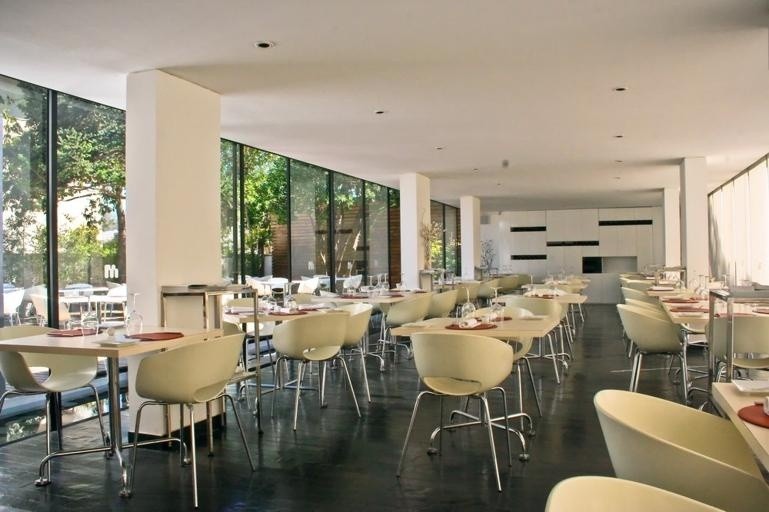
[
  {"x1": 651, "y1": 265, "x2": 755, "y2": 313},
  {"x1": 255, "y1": 275, "x2": 416, "y2": 315},
  {"x1": 66, "y1": 289, "x2": 145, "y2": 338},
  {"x1": 440, "y1": 267, "x2": 573, "y2": 324}
]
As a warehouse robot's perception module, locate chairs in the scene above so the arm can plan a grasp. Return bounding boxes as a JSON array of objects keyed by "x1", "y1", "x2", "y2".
[
  {"x1": 592, "y1": 388, "x2": 767, "y2": 511},
  {"x1": 543, "y1": 474, "x2": 721, "y2": 511},
  {"x1": 616, "y1": 270, "x2": 768, "y2": 415},
  {"x1": 1, "y1": 278, "x2": 256, "y2": 511},
  {"x1": 221, "y1": 270, "x2": 590, "y2": 432},
  {"x1": 397, "y1": 331, "x2": 514, "y2": 491}
]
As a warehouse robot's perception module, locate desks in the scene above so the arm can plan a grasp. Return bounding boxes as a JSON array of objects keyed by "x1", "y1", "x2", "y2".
[
  {"x1": 395, "y1": 316, "x2": 560, "y2": 462},
  {"x1": 710, "y1": 382, "x2": 767, "y2": 467}
]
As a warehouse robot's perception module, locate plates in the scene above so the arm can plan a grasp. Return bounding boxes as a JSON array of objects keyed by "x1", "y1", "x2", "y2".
[
  {"x1": 732, "y1": 378, "x2": 768, "y2": 394},
  {"x1": 401, "y1": 322, "x2": 433, "y2": 330},
  {"x1": 94, "y1": 337, "x2": 139, "y2": 347}
]
